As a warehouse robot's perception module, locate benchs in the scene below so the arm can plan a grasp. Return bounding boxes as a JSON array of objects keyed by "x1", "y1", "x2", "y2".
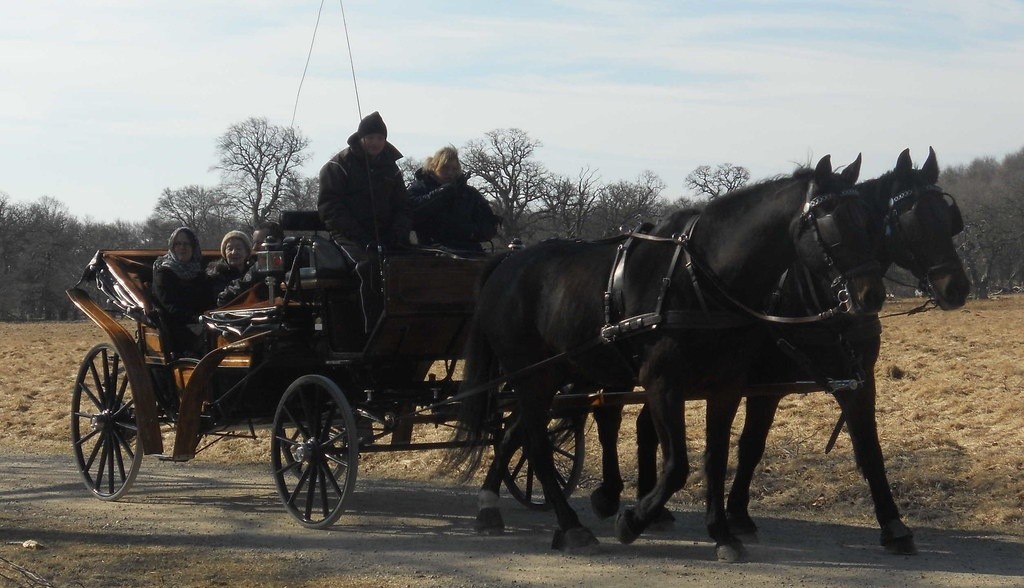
[
  {"x1": 280, "y1": 210, "x2": 502, "y2": 297},
  {"x1": 104, "y1": 250, "x2": 258, "y2": 363},
  {"x1": 199, "y1": 208, "x2": 332, "y2": 354}
]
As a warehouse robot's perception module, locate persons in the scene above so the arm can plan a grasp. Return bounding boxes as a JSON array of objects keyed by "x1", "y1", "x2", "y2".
[
  {"x1": 148, "y1": 223, "x2": 285, "y2": 358},
  {"x1": 317, "y1": 112, "x2": 498, "y2": 336}
]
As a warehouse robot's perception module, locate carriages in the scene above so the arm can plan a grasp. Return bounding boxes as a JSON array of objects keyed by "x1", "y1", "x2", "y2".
[{"x1": 66, "y1": 145, "x2": 971, "y2": 562}]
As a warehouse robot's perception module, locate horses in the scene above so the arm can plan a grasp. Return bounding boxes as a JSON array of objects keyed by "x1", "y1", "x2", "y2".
[{"x1": 427, "y1": 146, "x2": 972, "y2": 563}]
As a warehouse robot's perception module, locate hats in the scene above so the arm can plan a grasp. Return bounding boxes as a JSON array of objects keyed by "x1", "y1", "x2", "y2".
[
  {"x1": 358, "y1": 111, "x2": 387, "y2": 139},
  {"x1": 221, "y1": 230, "x2": 252, "y2": 258}
]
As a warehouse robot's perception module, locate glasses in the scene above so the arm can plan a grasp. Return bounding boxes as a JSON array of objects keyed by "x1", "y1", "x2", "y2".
[{"x1": 171, "y1": 240, "x2": 193, "y2": 246}]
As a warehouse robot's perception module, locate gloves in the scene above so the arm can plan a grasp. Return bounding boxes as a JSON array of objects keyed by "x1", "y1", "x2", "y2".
[{"x1": 442, "y1": 182, "x2": 457, "y2": 196}]
